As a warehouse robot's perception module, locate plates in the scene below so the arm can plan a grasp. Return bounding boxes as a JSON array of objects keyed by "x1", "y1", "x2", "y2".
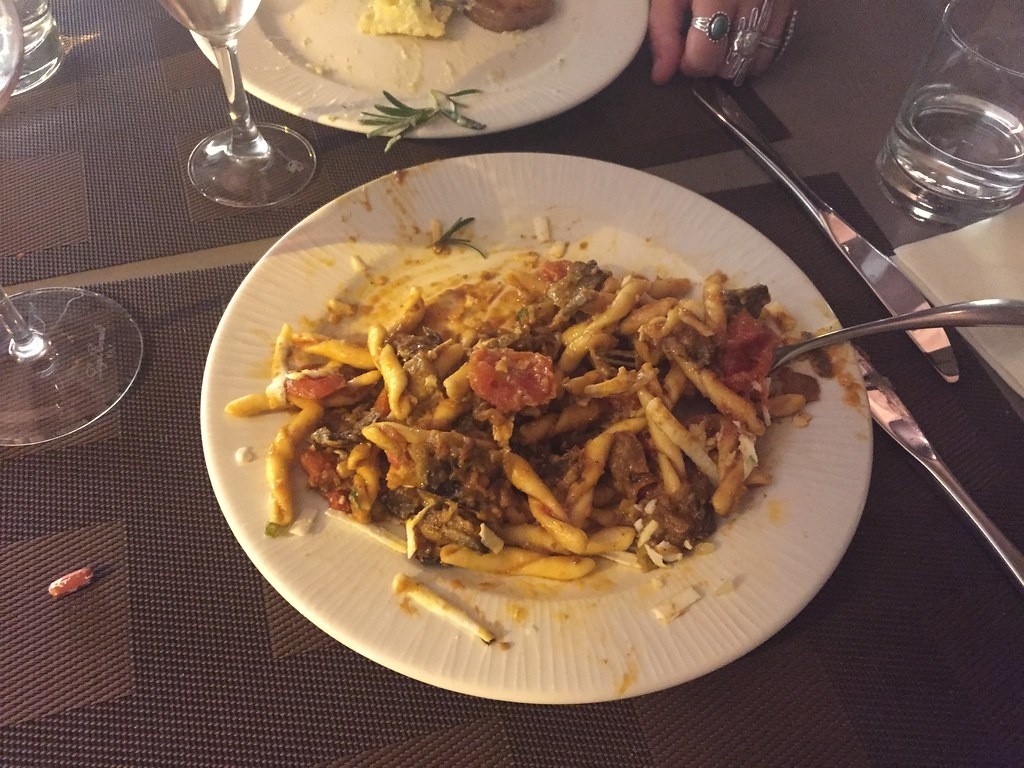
[
  {"x1": 197, "y1": 152, "x2": 876, "y2": 705},
  {"x1": 189, "y1": 1, "x2": 651, "y2": 140}
]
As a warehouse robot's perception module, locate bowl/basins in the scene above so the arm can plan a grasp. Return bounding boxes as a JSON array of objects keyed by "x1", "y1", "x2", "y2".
[{"x1": 0, "y1": 1, "x2": 24, "y2": 116}]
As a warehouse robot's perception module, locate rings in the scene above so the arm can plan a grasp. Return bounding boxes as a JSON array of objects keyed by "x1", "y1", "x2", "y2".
[
  {"x1": 690, "y1": 11, "x2": 732, "y2": 44},
  {"x1": 726, "y1": 1, "x2": 774, "y2": 87},
  {"x1": 762, "y1": 35, "x2": 781, "y2": 51}
]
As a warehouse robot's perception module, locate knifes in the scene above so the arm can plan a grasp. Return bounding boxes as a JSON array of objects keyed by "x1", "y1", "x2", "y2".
[
  {"x1": 874, "y1": 0, "x2": 1024, "y2": 230},
  {"x1": 851, "y1": 344, "x2": 1023, "y2": 588},
  {"x1": 693, "y1": 80, "x2": 960, "y2": 384}
]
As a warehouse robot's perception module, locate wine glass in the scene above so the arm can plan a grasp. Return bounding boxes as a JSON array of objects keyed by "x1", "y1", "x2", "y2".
[
  {"x1": 155, "y1": 0, "x2": 318, "y2": 207},
  {"x1": 0, "y1": 285, "x2": 144, "y2": 447}
]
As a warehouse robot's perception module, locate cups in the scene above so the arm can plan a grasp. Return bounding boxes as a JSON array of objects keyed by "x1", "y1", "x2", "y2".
[{"x1": 8, "y1": 0, "x2": 65, "y2": 97}]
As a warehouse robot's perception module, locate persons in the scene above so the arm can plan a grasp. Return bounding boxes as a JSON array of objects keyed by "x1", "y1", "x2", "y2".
[{"x1": 648, "y1": 1, "x2": 795, "y2": 85}]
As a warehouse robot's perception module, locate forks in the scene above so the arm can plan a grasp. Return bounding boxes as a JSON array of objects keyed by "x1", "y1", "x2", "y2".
[{"x1": 594, "y1": 294, "x2": 1024, "y2": 379}]
